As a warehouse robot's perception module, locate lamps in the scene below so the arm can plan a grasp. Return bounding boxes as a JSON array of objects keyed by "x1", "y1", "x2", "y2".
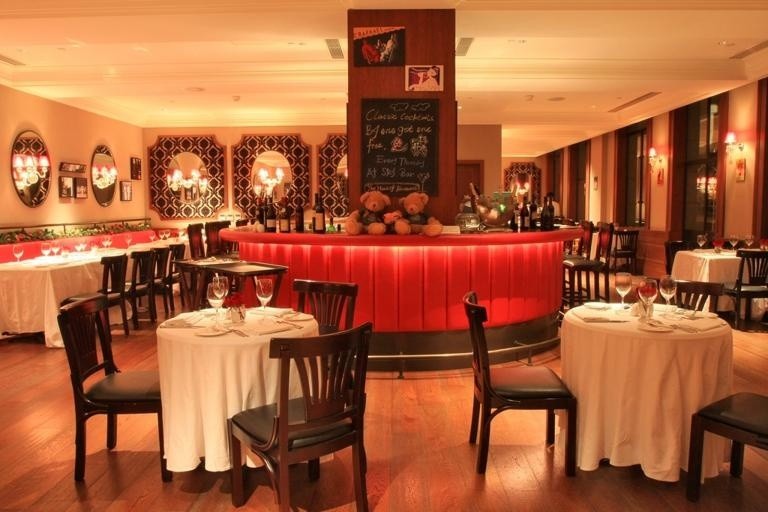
[
  {"x1": 11, "y1": 153, "x2": 50, "y2": 190},
  {"x1": 647, "y1": 147, "x2": 665, "y2": 184},
  {"x1": 724, "y1": 132, "x2": 746, "y2": 182}
]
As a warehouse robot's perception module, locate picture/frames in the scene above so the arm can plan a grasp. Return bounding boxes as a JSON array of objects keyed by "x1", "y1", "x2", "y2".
[
  {"x1": 404, "y1": 65, "x2": 445, "y2": 92},
  {"x1": 120, "y1": 158, "x2": 142, "y2": 201},
  {"x1": 58, "y1": 162, "x2": 89, "y2": 199}
]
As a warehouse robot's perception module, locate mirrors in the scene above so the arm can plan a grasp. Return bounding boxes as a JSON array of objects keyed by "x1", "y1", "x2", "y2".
[
  {"x1": 90, "y1": 144, "x2": 117, "y2": 206},
  {"x1": 504, "y1": 161, "x2": 541, "y2": 209},
  {"x1": 147, "y1": 135, "x2": 228, "y2": 220},
  {"x1": 10, "y1": 130, "x2": 52, "y2": 208},
  {"x1": 231, "y1": 133, "x2": 313, "y2": 222},
  {"x1": 316, "y1": 133, "x2": 348, "y2": 219}
]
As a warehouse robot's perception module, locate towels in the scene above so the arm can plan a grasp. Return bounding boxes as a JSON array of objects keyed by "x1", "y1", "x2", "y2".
[
  {"x1": 681, "y1": 318, "x2": 725, "y2": 333},
  {"x1": 572, "y1": 309, "x2": 608, "y2": 322}
]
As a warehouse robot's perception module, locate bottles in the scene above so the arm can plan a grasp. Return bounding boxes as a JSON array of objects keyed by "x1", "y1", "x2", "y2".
[
  {"x1": 548, "y1": 194, "x2": 555, "y2": 230},
  {"x1": 314, "y1": 196, "x2": 325, "y2": 234},
  {"x1": 279, "y1": 196, "x2": 291, "y2": 233},
  {"x1": 295, "y1": 197, "x2": 304, "y2": 232},
  {"x1": 327, "y1": 216, "x2": 336, "y2": 232},
  {"x1": 541, "y1": 195, "x2": 549, "y2": 229},
  {"x1": 510, "y1": 207, "x2": 518, "y2": 230},
  {"x1": 520, "y1": 196, "x2": 530, "y2": 231},
  {"x1": 255, "y1": 198, "x2": 264, "y2": 223},
  {"x1": 265, "y1": 197, "x2": 276, "y2": 233},
  {"x1": 529, "y1": 197, "x2": 537, "y2": 230},
  {"x1": 312, "y1": 193, "x2": 319, "y2": 233}
]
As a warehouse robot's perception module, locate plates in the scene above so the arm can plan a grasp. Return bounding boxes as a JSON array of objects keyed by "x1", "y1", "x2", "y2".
[
  {"x1": 638, "y1": 326, "x2": 671, "y2": 332},
  {"x1": 198, "y1": 325, "x2": 231, "y2": 337},
  {"x1": 287, "y1": 313, "x2": 313, "y2": 322},
  {"x1": 583, "y1": 301, "x2": 610, "y2": 309}
]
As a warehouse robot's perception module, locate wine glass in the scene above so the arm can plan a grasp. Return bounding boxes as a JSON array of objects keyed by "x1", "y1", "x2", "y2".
[
  {"x1": 74, "y1": 240, "x2": 82, "y2": 253},
  {"x1": 697, "y1": 234, "x2": 706, "y2": 249},
  {"x1": 159, "y1": 230, "x2": 165, "y2": 240},
  {"x1": 102, "y1": 238, "x2": 109, "y2": 248},
  {"x1": 12, "y1": 245, "x2": 24, "y2": 261},
  {"x1": 149, "y1": 231, "x2": 155, "y2": 241},
  {"x1": 206, "y1": 282, "x2": 226, "y2": 315},
  {"x1": 80, "y1": 240, "x2": 88, "y2": 250},
  {"x1": 659, "y1": 276, "x2": 677, "y2": 311},
  {"x1": 212, "y1": 277, "x2": 229, "y2": 300},
  {"x1": 41, "y1": 243, "x2": 52, "y2": 256},
  {"x1": 51, "y1": 241, "x2": 60, "y2": 256},
  {"x1": 615, "y1": 273, "x2": 632, "y2": 306},
  {"x1": 107, "y1": 237, "x2": 112, "y2": 248},
  {"x1": 164, "y1": 230, "x2": 170, "y2": 241},
  {"x1": 638, "y1": 279, "x2": 658, "y2": 323},
  {"x1": 745, "y1": 234, "x2": 754, "y2": 248},
  {"x1": 255, "y1": 278, "x2": 273, "y2": 309},
  {"x1": 124, "y1": 235, "x2": 132, "y2": 246},
  {"x1": 729, "y1": 232, "x2": 739, "y2": 255}
]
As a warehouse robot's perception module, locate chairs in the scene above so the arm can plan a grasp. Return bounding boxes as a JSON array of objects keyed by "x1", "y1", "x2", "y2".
[
  {"x1": 553, "y1": 216, "x2": 768, "y2": 327},
  {"x1": 686, "y1": 391, "x2": 768, "y2": 502},
  {"x1": 462, "y1": 291, "x2": 576, "y2": 477},
  {"x1": 292, "y1": 279, "x2": 358, "y2": 338},
  {"x1": 226, "y1": 322, "x2": 372, "y2": 511}
]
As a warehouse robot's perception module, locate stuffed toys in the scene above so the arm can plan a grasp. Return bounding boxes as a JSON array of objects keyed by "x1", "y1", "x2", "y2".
[
  {"x1": 392, "y1": 192, "x2": 443, "y2": 237},
  {"x1": 345, "y1": 191, "x2": 392, "y2": 235}
]
